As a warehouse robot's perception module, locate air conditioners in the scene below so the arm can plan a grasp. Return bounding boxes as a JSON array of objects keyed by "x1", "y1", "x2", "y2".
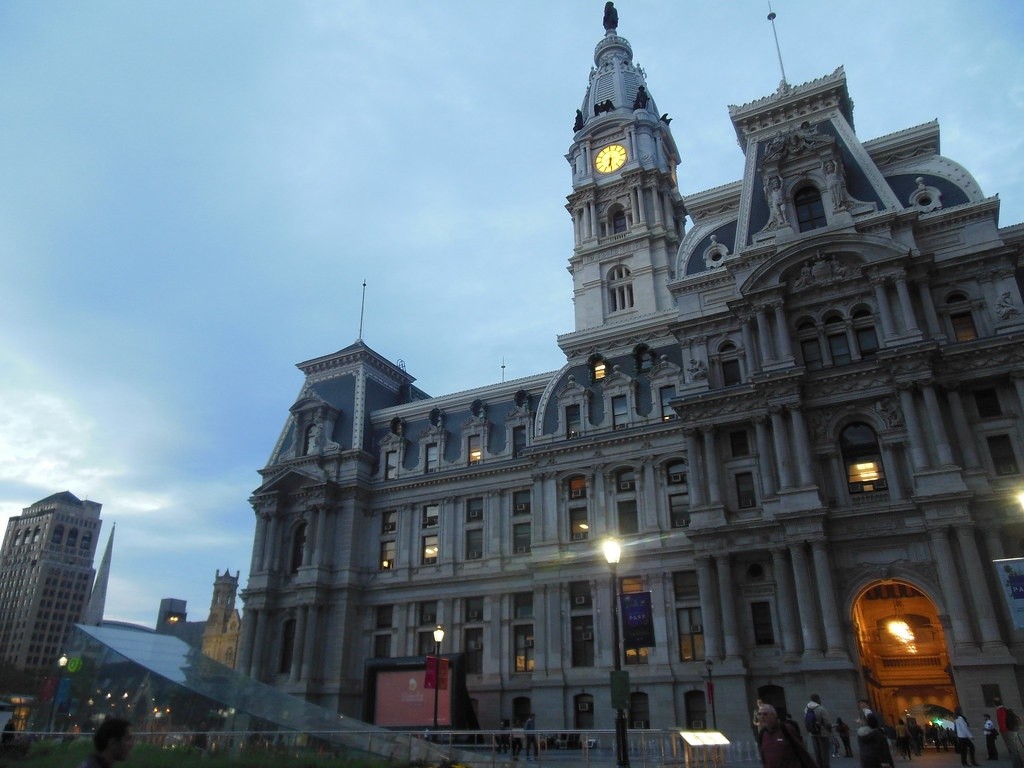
[
  {"x1": 517, "y1": 451, "x2": 525, "y2": 457},
  {"x1": 516, "y1": 546, "x2": 525, "y2": 553},
  {"x1": 576, "y1": 596, "x2": 584, "y2": 603},
  {"x1": 676, "y1": 519, "x2": 685, "y2": 527},
  {"x1": 423, "y1": 614, "x2": 432, "y2": 622},
  {"x1": 424, "y1": 517, "x2": 431, "y2": 522},
  {"x1": 742, "y1": 499, "x2": 752, "y2": 507},
  {"x1": 873, "y1": 479, "x2": 888, "y2": 490},
  {"x1": 525, "y1": 639, "x2": 533, "y2": 647},
  {"x1": 470, "y1": 510, "x2": 479, "y2": 517},
  {"x1": 573, "y1": 489, "x2": 582, "y2": 498},
  {"x1": 588, "y1": 740, "x2": 595, "y2": 748},
  {"x1": 690, "y1": 625, "x2": 702, "y2": 633},
  {"x1": 469, "y1": 552, "x2": 477, "y2": 558},
  {"x1": 469, "y1": 611, "x2": 478, "y2": 618},
  {"x1": 634, "y1": 720, "x2": 647, "y2": 729},
  {"x1": 428, "y1": 468, "x2": 435, "y2": 472},
  {"x1": 571, "y1": 431, "x2": 580, "y2": 438},
  {"x1": 579, "y1": 703, "x2": 590, "y2": 711},
  {"x1": 472, "y1": 460, "x2": 479, "y2": 465},
  {"x1": 384, "y1": 524, "x2": 393, "y2": 530},
  {"x1": 425, "y1": 645, "x2": 434, "y2": 653},
  {"x1": 849, "y1": 483, "x2": 862, "y2": 493},
  {"x1": 619, "y1": 423, "x2": 628, "y2": 430},
  {"x1": 669, "y1": 414, "x2": 678, "y2": 419},
  {"x1": 517, "y1": 503, "x2": 526, "y2": 510},
  {"x1": 621, "y1": 481, "x2": 631, "y2": 490},
  {"x1": 473, "y1": 642, "x2": 481, "y2": 649},
  {"x1": 1000, "y1": 465, "x2": 1015, "y2": 475},
  {"x1": 573, "y1": 532, "x2": 584, "y2": 539},
  {"x1": 582, "y1": 632, "x2": 592, "y2": 641},
  {"x1": 672, "y1": 474, "x2": 682, "y2": 481},
  {"x1": 424, "y1": 557, "x2": 433, "y2": 563}
]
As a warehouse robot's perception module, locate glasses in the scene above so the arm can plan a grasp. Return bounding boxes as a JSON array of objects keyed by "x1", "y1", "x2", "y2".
[{"x1": 757, "y1": 712, "x2": 774, "y2": 716}]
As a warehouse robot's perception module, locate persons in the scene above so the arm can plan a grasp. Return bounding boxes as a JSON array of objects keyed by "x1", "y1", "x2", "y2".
[
  {"x1": 955, "y1": 708, "x2": 982, "y2": 767},
  {"x1": 753, "y1": 698, "x2": 800, "y2": 736},
  {"x1": 857, "y1": 713, "x2": 895, "y2": 768},
  {"x1": 821, "y1": 160, "x2": 844, "y2": 208},
  {"x1": 832, "y1": 717, "x2": 853, "y2": 758},
  {"x1": 498, "y1": 722, "x2": 507, "y2": 753},
  {"x1": 880, "y1": 718, "x2": 955, "y2": 761},
  {"x1": 424, "y1": 727, "x2": 429, "y2": 741},
  {"x1": 803, "y1": 694, "x2": 833, "y2": 768},
  {"x1": 510, "y1": 718, "x2": 523, "y2": 760},
  {"x1": 857, "y1": 700, "x2": 884, "y2": 727},
  {"x1": 758, "y1": 704, "x2": 818, "y2": 768},
  {"x1": 525, "y1": 713, "x2": 538, "y2": 761},
  {"x1": 78, "y1": 719, "x2": 133, "y2": 768},
  {"x1": 993, "y1": 697, "x2": 1024, "y2": 768},
  {"x1": 766, "y1": 173, "x2": 788, "y2": 225},
  {"x1": 982, "y1": 713, "x2": 998, "y2": 760},
  {"x1": 1, "y1": 718, "x2": 15, "y2": 745}
]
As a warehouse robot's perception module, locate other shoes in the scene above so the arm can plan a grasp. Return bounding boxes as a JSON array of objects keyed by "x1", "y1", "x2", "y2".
[
  {"x1": 962, "y1": 763, "x2": 971, "y2": 768},
  {"x1": 513, "y1": 758, "x2": 518, "y2": 761},
  {"x1": 971, "y1": 763, "x2": 980, "y2": 767},
  {"x1": 832, "y1": 754, "x2": 836, "y2": 758},
  {"x1": 836, "y1": 754, "x2": 840, "y2": 758},
  {"x1": 535, "y1": 757, "x2": 538, "y2": 760},
  {"x1": 527, "y1": 758, "x2": 532, "y2": 761}
]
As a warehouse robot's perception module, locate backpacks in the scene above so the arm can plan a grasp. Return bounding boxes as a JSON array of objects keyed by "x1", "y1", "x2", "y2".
[
  {"x1": 804, "y1": 704, "x2": 820, "y2": 733},
  {"x1": 1003, "y1": 707, "x2": 1018, "y2": 731}
]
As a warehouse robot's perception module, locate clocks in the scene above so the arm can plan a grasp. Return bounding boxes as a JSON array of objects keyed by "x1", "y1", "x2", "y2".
[{"x1": 594, "y1": 143, "x2": 627, "y2": 172}]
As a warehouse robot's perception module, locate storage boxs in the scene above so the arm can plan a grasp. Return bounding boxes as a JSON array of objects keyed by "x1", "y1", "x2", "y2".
[{"x1": 559, "y1": 734, "x2": 575, "y2": 750}]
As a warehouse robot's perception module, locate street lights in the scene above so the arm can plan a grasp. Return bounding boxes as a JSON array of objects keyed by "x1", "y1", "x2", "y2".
[
  {"x1": 431, "y1": 625, "x2": 445, "y2": 744},
  {"x1": 42, "y1": 652, "x2": 68, "y2": 741},
  {"x1": 599, "y1": 538, "x2": 631, "y2": 768}
]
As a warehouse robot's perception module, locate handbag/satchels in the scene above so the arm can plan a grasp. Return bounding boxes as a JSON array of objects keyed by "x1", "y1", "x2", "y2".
[
  {"x1": 990, "y1": 728, "x2": 1000, "y2": 737},
  {"x1": 954, "y1": 739, "x2": 963, "y2": 754}
]
